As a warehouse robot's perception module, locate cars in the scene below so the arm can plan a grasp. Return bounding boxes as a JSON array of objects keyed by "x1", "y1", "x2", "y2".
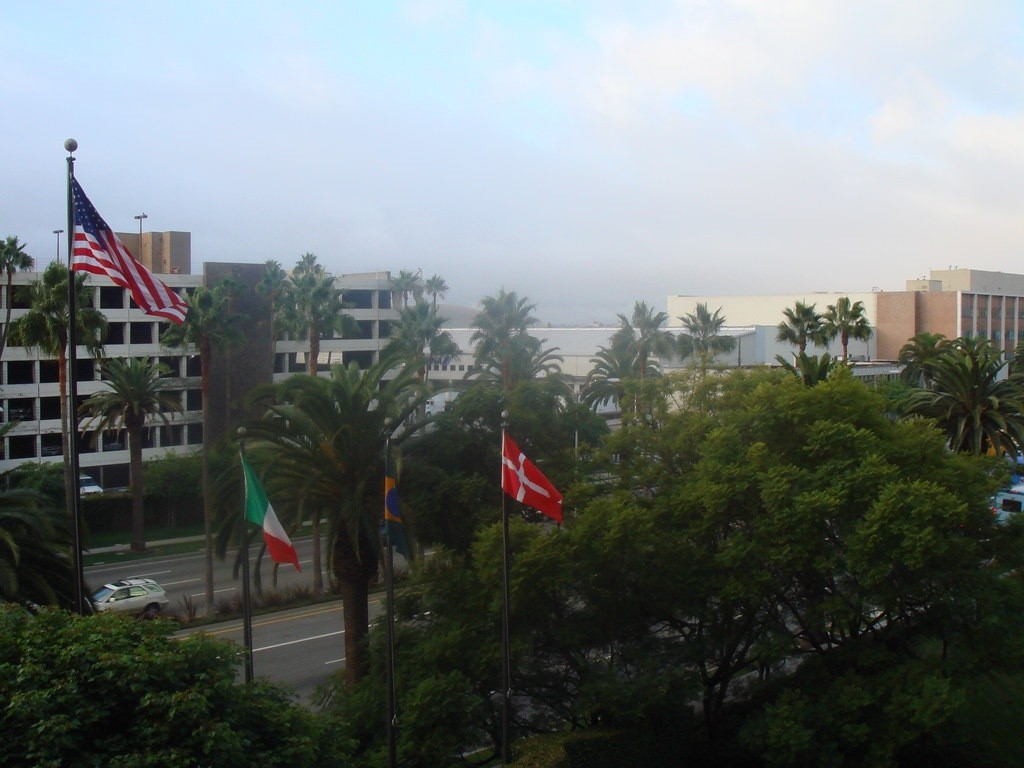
[{"x1": 1004, "y1": 449, "x2": 1024, "y2": 488}]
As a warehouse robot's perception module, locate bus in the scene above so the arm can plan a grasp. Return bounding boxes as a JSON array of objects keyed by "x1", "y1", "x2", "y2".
[{"x1": 992, "y1": 483, "x2": 1024, "y2": 530}]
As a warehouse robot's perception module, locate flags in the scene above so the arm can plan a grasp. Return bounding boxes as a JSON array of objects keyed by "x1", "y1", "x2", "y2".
[
  {"x1": 238, "y1": 449, "x2": 304, "y2": 574},
  {"x1": 501, "y1": 430, "x2": 564, "y2": 525},
  {"x1": 67, "y1": 157, "x2": 189, "y2": 330},
  {"x1": 384, "y1": 446, "x2": 415, "y2": 562}
]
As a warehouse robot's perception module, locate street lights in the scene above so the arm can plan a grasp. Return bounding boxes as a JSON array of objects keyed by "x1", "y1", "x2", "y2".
[
  {"x1": 53, "y1": 230, "x2": 64, "y2": 265},
  {"x1": 134, "y1": 212, "x2": 147, "y2": 264}
]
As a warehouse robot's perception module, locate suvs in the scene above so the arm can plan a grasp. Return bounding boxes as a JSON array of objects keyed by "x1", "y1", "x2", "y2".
[
  {"x1": 80, "y1": 474, "x2": 104, "y2": 501},
  {"x1": 91, "y1": 578, "x2": 170, "y2": 620}
]
